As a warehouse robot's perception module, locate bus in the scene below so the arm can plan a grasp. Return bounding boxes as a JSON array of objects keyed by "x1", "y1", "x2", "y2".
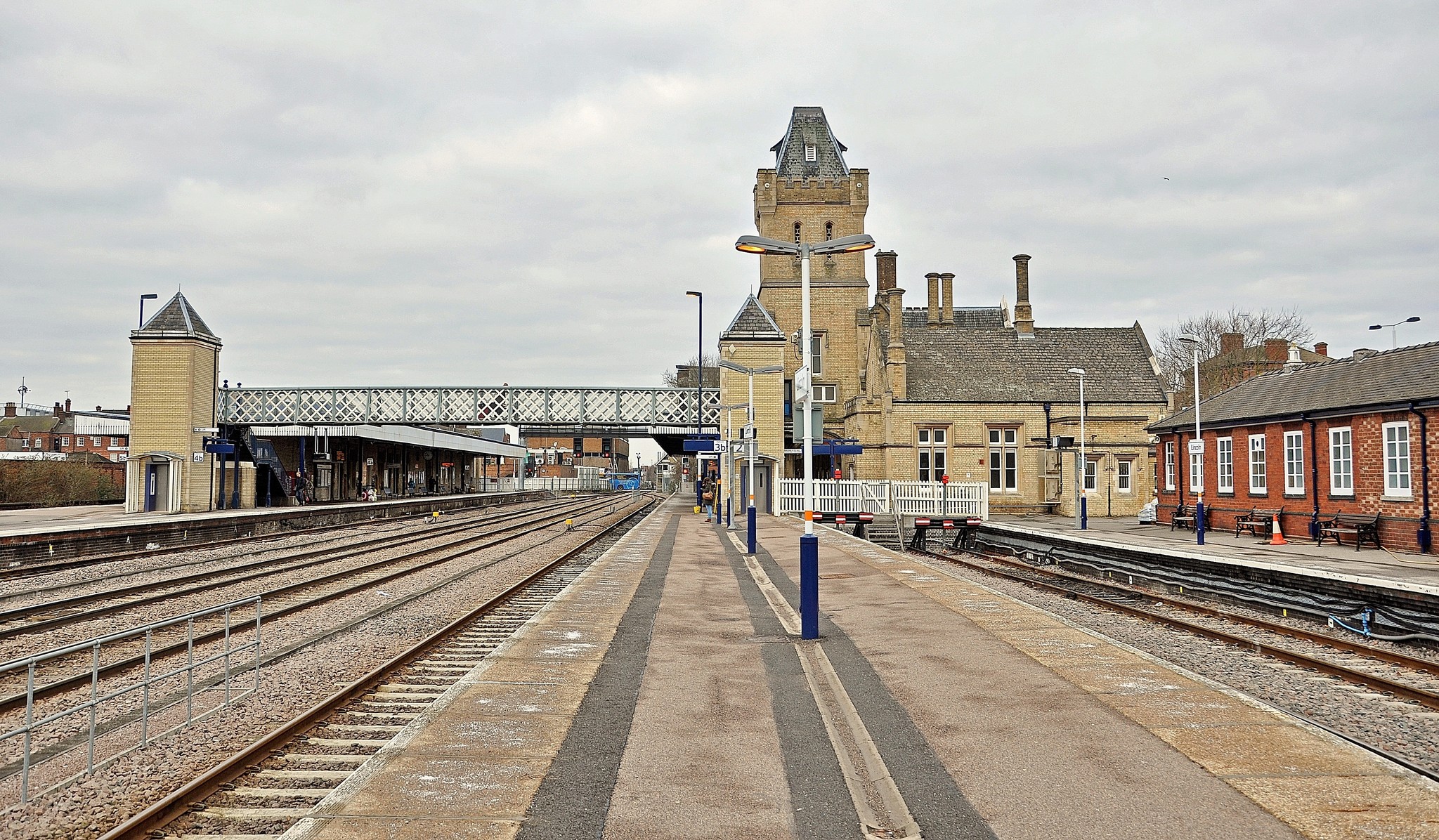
[{"x1": 603, "y1": 473, "x2": 640, "y2": 490}]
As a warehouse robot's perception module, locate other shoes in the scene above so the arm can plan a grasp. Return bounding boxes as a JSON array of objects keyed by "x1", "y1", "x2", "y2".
[{"x1": 299, "y1": 505, "x2": 303, "y2": 506}]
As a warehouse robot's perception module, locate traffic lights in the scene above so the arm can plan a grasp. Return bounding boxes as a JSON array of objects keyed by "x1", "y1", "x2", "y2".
[
  {"x1": 601, "y1": 438, "x2": 611, "y2": 458},
  {"x1": 526, "y1": 455, "x2": 534, "y2": 473},
  {"x1": 682, "y1": 457, "x2": 689, "y2": 474},
  {"x1": 573, "y1": 437, "x2": 584, "y2": 458}
]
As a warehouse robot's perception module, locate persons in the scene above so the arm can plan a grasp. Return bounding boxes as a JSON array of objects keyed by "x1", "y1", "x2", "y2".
[
  {"x1": 429, "y1": 475, "x2": 436, "y2": 494},
  {"x1": 701, "y1": 477, "x2": 714, "y2": 522},
  {"x1": 294, "y1": 472, "x2": 305, "y2": 506},
  {"x1": 305, "y1": 478, "x2": 314, "y2": 504},
  {"x1": 408, "y1": 479, "x2": 416, "y2": 498}
]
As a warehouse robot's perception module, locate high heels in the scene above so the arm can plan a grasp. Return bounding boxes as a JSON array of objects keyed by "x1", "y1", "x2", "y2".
[{"x1": 705, "y1": 519, "x2": 711, "y2": 523}]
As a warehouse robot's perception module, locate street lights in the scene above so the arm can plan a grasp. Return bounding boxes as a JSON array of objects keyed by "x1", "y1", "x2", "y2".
[
  {"x1": 636, "y1": 452, "x2": 641, "y2": 473},
  {"x1": 735, "y1": 233, "x2": 876, "y2": 641},
  {"x1": 716, "y1": 360, "x2": 784, "y2": 552},
  {"x1": 706, "y1": 403, "x2": 750, "y2": 529},
  {"x1": 1177, "y1": 334, "x2": 1205, "y2": 546},
  {"x1": 1090, "y1": 433, "x2": 1116, "y2": 518},
  {"x1": 1368, "y1": 316, "x2": 1421, "y2": 349},
  {"x1": 686, "y1": 290, "x2": 703, "y2": 512},
  {"x1": 1066, "y1": 368, "x2": 1089, "y2": 529}
]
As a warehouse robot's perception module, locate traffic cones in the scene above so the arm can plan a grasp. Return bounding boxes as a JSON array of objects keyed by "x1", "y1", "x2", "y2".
[{"x1": 1269, "y1": 514, "x2": 1287, "y2": 545}]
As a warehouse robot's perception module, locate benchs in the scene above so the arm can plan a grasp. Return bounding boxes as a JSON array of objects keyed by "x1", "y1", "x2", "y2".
[
  {"x1": 384, "y1": 487, "x2": 399, "y2": 500},
  {"x1": 407, "y1": 487, "x2": 421, "y2": 498},
  {"x1": 420, "y1": 487, "x2": 433, "y2": 497},
  {"x1": 464, "y1": 484, "x2": 476, "y2": 494},
  {"x1": 1169, "y1": 502, "x2": 1213, "y2": 534},
  {"x1": 440, "y1": 486, "x2": 452, "y2": 496},
  {"x1": 1313, "y1": 509, "x2": 1385, "y2": 552},
  {"x1": 1232, "y1": 505, "x2": 1285, "y2": 542}
]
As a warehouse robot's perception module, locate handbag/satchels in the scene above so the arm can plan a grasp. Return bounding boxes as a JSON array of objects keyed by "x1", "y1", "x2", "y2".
[
  {"x1": 703, "y1": 492, "x2": 713, "y2": 500},
  {"x1": 405, "y1": 486, "x2": 409, "y2": 490},
  {"x1": 413, "y1": 486, "x2": 416, "y2": 489}
]
